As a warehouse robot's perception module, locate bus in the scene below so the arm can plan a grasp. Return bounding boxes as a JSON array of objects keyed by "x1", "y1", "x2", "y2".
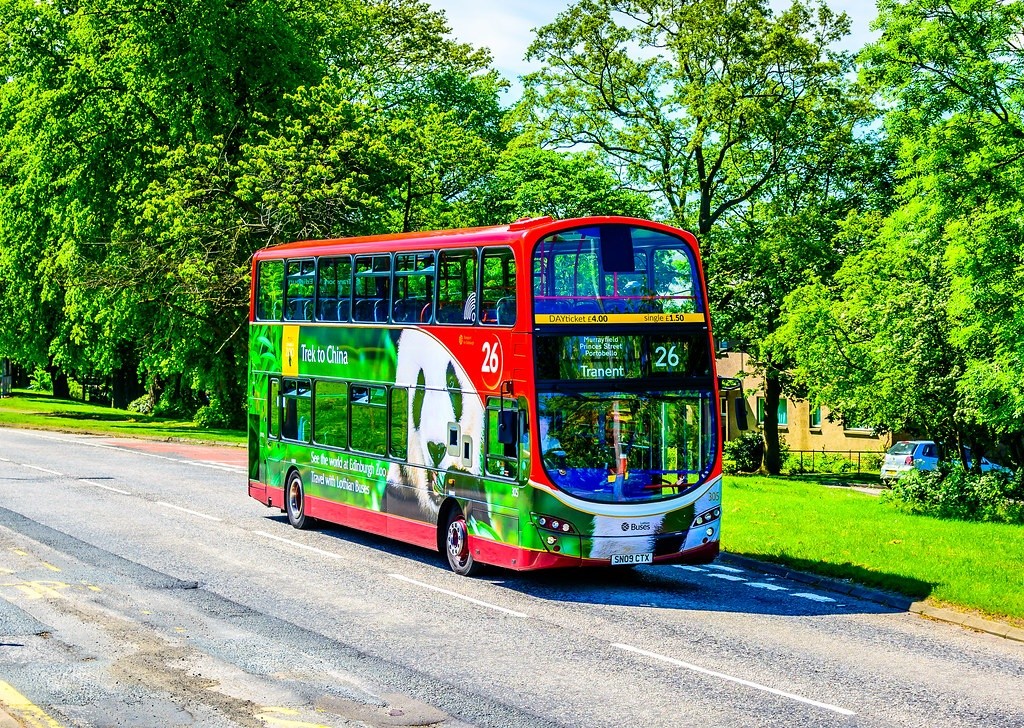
[{"x1": 247, "y1": 217, "x2": 748, "y2": 576}]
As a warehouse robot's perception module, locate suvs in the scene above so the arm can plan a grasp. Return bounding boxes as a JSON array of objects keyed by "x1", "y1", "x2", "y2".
[{"x1": 880, "y1": 440, "x2": 1014, "y2": 489}]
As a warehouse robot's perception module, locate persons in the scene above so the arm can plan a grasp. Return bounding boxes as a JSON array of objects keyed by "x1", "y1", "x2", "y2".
[{"x1": 523, "y1": 416, "x2": 574, "y2": 472}]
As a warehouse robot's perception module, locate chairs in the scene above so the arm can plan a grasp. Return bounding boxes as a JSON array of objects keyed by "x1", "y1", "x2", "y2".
[{"x1": 284, "y1": 296, "x2": 663, "y2": 324}]
